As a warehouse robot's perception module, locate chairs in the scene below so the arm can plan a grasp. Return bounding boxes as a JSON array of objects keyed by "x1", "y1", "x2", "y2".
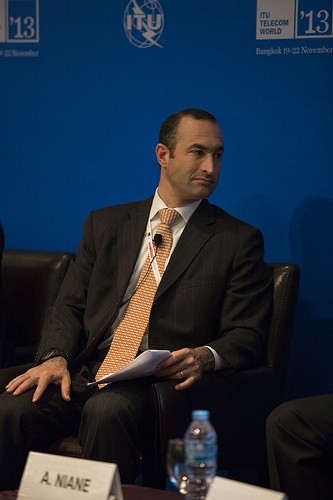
[{"x1": 0, "y1": 250, "x2": 302, "y2": 492}]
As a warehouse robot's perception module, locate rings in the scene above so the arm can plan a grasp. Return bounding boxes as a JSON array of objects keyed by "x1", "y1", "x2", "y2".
[{"x1": 180, "y1": 371, "x2": 185, "y2": 378}]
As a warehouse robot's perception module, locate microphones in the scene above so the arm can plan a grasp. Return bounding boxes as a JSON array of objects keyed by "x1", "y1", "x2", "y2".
[{"x1": 153, "y1": 234, "x2": 162, "y2": 244}]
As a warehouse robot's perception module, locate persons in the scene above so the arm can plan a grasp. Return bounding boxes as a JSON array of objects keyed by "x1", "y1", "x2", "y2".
[
  {"x1": 0, "y1": 108, "x2": 273, "y2": 492},
  {"x1": 265, "y1": 394, "x2": 333, "y2": 500}
]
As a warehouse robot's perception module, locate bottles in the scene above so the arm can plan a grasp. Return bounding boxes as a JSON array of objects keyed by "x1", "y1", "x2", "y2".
[{"x1": 183, "y1": 410, "x2": 216, "y2": 485}]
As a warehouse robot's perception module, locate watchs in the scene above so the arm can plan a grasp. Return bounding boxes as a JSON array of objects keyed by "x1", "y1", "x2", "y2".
[{"x1": 40, "y1": 348, "x2": 69, "y2": 363}]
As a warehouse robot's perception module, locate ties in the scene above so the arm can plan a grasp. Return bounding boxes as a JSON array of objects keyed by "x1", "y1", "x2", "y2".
[{"x1": 94, "y1": 208, "x2": 182, "y2": 390}]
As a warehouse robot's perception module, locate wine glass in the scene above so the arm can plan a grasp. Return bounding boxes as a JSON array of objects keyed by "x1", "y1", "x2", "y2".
[{"x1": 167, "y1": 440, "x2": 216, "y2": 500}]
